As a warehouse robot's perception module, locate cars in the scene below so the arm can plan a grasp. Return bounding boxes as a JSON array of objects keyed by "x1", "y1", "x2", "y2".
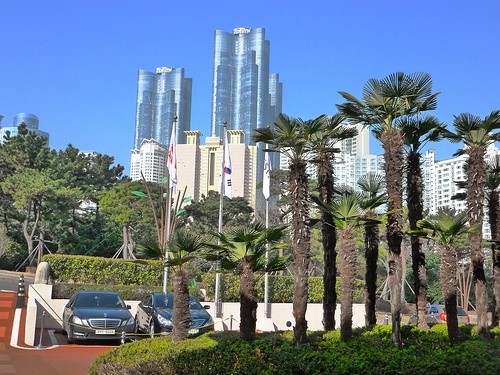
[
  {"x1": 408, "y1": 304, "x2": 470, "y2": 327},
  {"x1": 62, "y1": 291, "x2": 136, "y2": 344},
  {"x1": 135, "y1": 292, "x2": 214, "y2": 338}
]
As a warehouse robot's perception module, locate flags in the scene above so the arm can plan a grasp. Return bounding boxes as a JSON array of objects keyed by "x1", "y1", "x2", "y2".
[
  {"x1": 166, "y1": 120, "x2": 179, "y2": 188},
  {"x1": 223, "y1": 129, "x2": 233, "y2": 199},
  {"x1": 262, "y1": 152, "x2": 272, "y2": 200}
]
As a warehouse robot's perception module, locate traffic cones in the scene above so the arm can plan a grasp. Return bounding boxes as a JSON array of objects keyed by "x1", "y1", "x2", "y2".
[{"x1": 15, "y1": 275, "x2": 26, "y2": 308}]
